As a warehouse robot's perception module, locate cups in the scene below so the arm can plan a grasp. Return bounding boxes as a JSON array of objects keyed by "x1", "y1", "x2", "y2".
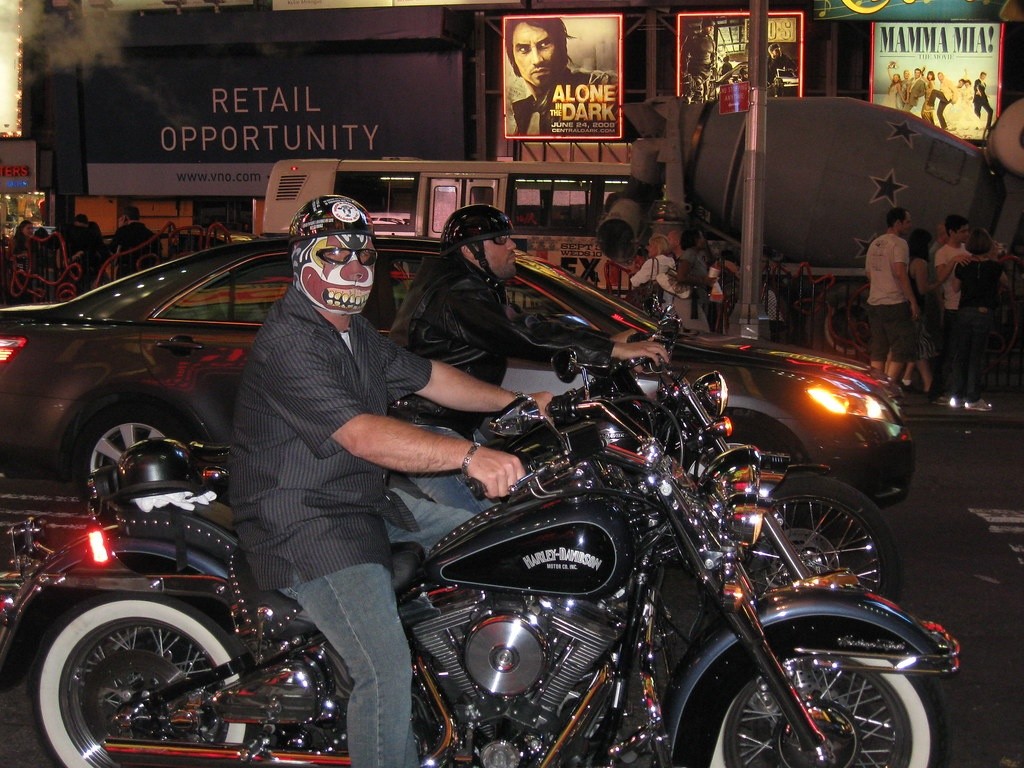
[{"x1": 708, "y1": 266, "x2": 721, "y2": 287}]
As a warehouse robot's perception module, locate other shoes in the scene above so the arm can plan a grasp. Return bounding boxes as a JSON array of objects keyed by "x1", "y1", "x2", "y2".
[{"x1": 965, "y1": 399, "x2": 993, "y2": 412}]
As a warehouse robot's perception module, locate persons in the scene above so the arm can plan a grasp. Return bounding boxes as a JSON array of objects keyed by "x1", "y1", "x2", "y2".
[
  {"x1": 226, "y1": 193, "x2": 556, "y2": 768},
  {"x1": 630, "y1": 228, "x2": 739, "y2": 334},
  {"x1": 384, "y1": 205, "x2": 670, "y2": 515},
  {"x1": 7, "y1": 206, "x2": 162, "y2": 306},
  {"x1": 681, "y1": 18, "x2": 797, "y2": 103},
  {"x1": 887, "y1": 61, "x2": 993, "y2": 131},
  {"x1": 765, "y1": 250, "x2": 790, "y2": 342},
  {"x1": 506, "y1": 20, "x2": 616, "y2": 137},
  {"x1": 865, "y1": 206, "x2": 1008, "y2": 412}
]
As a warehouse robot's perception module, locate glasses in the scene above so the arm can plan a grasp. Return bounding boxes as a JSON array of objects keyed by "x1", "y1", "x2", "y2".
[
  {"x1": 489, "y1": 235, "x2": 510, "y2": 245},
  {"x1": 316, "y1": 248, "x2": 376, "y2": 266}
]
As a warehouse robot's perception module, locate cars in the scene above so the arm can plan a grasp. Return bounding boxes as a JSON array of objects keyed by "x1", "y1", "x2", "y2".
[{"x1": 0, "y1": 234, "x2": 918, "y2": 514}]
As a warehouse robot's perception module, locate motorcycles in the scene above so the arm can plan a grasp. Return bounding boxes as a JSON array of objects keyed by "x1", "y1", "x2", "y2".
[
  {"x1": 82, "y1": 294, "x2": 905, "y2": 607},
  {"x1": 0, "y1": 345, "x2": 963, "y2": 768}
]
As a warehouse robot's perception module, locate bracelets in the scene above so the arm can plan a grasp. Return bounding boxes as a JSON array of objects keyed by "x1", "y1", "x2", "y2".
[
  {"x1": 514, "y1": 391, "x2": 533, "y2": 402},
  {"x1": 462, "y1": 441, "x2": 481, "y2": 476}
]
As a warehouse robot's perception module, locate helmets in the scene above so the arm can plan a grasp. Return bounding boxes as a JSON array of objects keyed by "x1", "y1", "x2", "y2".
[
  {"x1": 291, "y1": 193, "x2": 377, "y2": 315},
  {"x1": 439, "y1": 203, "x2": 514, "y2": 254}
]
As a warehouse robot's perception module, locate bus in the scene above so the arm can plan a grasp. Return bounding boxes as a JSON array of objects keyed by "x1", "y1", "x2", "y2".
[{"x1": 259, "y1": 157, "x2": 672, "y2": 300}]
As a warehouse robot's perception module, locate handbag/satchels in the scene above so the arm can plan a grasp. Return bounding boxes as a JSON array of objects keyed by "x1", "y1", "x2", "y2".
[{"x1": 626, "y1": 280, "x2": 664, "y2": 313}]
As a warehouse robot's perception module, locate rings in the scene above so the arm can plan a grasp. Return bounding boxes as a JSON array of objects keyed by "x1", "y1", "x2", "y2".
[{"x1": 916, "y1": 314, "x2": 918, "y2": 317}]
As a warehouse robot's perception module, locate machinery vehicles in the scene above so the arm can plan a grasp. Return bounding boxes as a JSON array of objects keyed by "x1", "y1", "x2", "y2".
[{"x1": 593, "y1": 91, "x2": 1024, "y2": 319}]
